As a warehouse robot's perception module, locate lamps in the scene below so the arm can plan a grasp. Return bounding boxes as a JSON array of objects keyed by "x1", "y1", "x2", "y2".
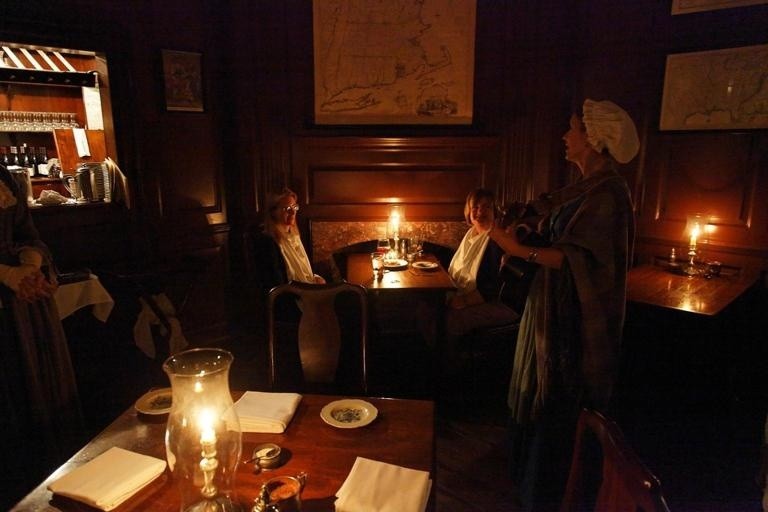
[
  {"x1": 383, "y1": 205, "x2": 411, "y2": 271},
  {"x1": 678, "y1": 214, "x2": 707, "y2": 277}
]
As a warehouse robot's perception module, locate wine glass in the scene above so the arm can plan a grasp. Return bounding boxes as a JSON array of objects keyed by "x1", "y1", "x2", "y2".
[{"x1": 376, "y1": 238, "x2": 390, "y2": 257}]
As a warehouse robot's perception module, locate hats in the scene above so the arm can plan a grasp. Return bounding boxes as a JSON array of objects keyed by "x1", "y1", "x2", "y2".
[{"x1": 583, "y1": 99, "x2": 640, "y2": 165}]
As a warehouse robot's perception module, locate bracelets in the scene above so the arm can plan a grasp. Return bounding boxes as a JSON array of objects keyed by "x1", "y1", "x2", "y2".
[{"x1": 524, "y1": 246, "x2": 544, "y2": 263}]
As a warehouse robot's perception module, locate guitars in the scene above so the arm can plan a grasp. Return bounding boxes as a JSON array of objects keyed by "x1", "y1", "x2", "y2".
[{"x1": 497, "y1": 204, "x2": 546, "y2": 316}]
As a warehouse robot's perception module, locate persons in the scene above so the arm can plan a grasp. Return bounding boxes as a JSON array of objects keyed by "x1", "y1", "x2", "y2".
[
  {"x1": 2, "y1": 162, "x2": 78, "y2": 483},
  {"x1": 489, "y1": 99, "x2": 641, "y2": 509},
  {"x1": 243, "y1": 185, "x2": 328, "y2": 325},
  {"x1": 446, "y1": 187, "x2": 527, "y2": 375}
]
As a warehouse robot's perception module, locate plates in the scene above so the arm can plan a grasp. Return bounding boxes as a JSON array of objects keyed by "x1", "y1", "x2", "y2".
[
  {"x1": 319, "y1": 399, "x2": 378, "y2": 430},
  {"x1": 134, "y1": 388, "x2": 172, "y2": 416},
  {"x1": 383, "y1": 259, "x2": 408, "y2": 269},
  {"x1": 411, "y1": 260, "x2": 437, "y2": 270}
]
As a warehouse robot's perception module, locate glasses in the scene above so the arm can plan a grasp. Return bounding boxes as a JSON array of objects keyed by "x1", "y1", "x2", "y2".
[{"x1": 274, "y1": 204, "x2": 300, "y2": 212}]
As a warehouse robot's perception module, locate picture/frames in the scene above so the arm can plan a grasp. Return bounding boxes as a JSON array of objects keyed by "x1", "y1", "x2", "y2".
[
  {"x1": 651, "y1": 44, "x2": 768, "y2": 137},
  {"x1": 668, "y1": 0, "x2": 767, "y2": 21},
  {"x1": 155, "y1": 42, "x2": 209, "y2": 118}
]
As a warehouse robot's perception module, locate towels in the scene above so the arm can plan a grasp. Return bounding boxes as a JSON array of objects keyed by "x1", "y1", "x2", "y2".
[
  {"x1": 262, "y1": 220, "x2": 318, "y2": 286},
  {"x1": 446, "y1": 222, "x2": 496, "y2": 296}
]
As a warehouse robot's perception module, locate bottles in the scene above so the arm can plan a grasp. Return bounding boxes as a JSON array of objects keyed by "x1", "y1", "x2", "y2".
[
  {"x1": 0, "y1": 144, "x2": 49, "y2": 177},
  {"x1": 0, "y1": 112, "x2": 80, "y2": 131},
  {"x1": 399, "y1": 239, "x2": 406, "y2": 256}
]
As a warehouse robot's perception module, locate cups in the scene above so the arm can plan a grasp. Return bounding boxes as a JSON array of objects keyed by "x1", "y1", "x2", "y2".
[
  {"x1": 406, "y1": 231, "x2": 425, "y2": 254},
  {"x1": 370, "y1": 251, "x2": 383, "y2": 278}
]
